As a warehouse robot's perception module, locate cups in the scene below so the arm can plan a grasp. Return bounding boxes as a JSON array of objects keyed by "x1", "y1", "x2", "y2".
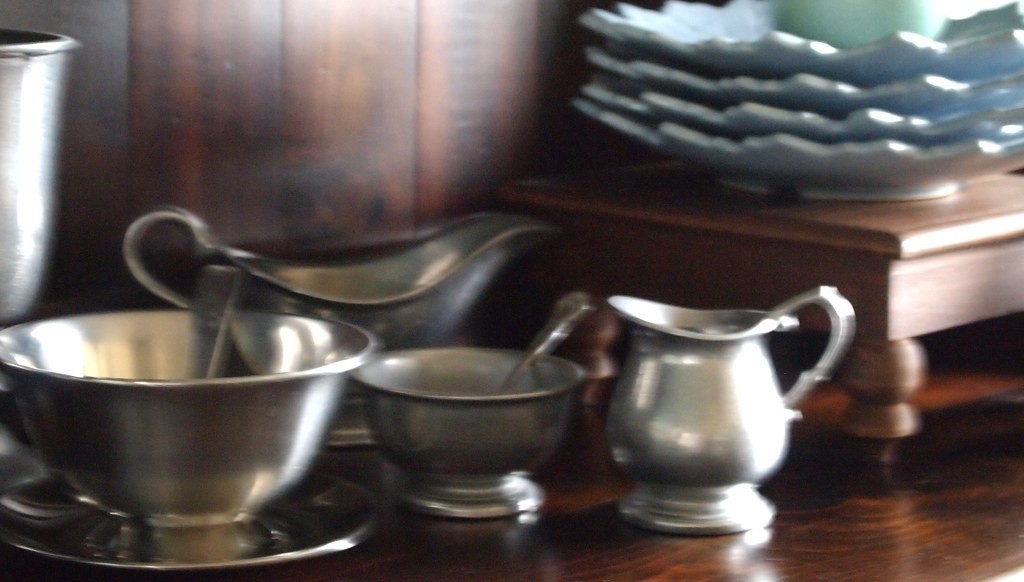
[{"x1": 0, "y1": 30, "x2": 80, "y2": 323}]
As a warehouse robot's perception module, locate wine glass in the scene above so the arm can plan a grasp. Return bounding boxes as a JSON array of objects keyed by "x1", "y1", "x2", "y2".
[{"x1": 351, "y1": 345, "x2": 588, "y2": 518}]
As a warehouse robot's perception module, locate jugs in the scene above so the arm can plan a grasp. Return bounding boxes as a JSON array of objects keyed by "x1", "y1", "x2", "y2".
[
  {"x1": 124, "y1": 206, "x2": 551, "y2": 447},
  {"x1": 605, "y1": 284, "x2": 856, "y2": 536}
]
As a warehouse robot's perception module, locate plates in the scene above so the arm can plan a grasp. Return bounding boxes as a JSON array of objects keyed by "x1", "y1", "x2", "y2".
[
  {"x1": 0, "y1": 465, "x2": 379, "y2": 571},
  {"x1": 574, "y1": 0, "x2": 1024, "y2": 200}
]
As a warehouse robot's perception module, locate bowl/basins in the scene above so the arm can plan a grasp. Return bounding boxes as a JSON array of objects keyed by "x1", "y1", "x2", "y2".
[{"x1": 0, "y1": 309, "x2": 382, "y2": 526}]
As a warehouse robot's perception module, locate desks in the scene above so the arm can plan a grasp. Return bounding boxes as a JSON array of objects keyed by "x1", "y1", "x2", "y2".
[{"x1": 490, "y1": 166, "x2": 1024, "y2": 438}]
